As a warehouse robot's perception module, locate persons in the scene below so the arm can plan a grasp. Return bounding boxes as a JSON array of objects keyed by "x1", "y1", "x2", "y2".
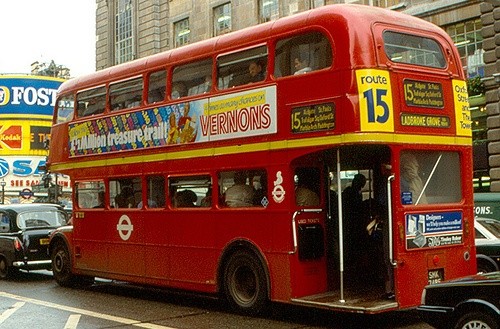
[
  {"x1": 116, "y1": 188, "x2": 164, "y2": 208},
  {"x1": 92, "y1": 191, "x2": 115, "y2": 209},
  {"x1": 248, "y1": 61, "x2": 266, "y2": 83},
  {"x1": 294, "y1": 169, "x2": 320, "y2": 206},
  {"x1": 173, "y1": 190, "x2": 198, "y2": 208},
  {"x1": 341, "y1": 175, "x2": 367, "y2": 268},
  {"x1": 167, "y1": 112, "x2": 177, "y2": 144},
  {"x1": 177, "y1": 102, "x2": 197, "y2": 133},
  {"x1": 293, "y1": 52, "x2": 312, "y2": 75},
  {"x1": 224, "y1": 171, "x2": 257, "y2": 207},
  {"x1": 200, "y1": 183, "x2": 224, "y2": 207}
]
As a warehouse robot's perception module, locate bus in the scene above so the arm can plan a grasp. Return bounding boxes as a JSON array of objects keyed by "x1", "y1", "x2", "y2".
[{"x1": 46, "y1": 4, "x2": 477, "y2": 318}]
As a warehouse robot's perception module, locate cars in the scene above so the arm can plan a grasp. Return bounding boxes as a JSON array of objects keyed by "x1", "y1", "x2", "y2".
[
  {"x1": 1, "y1": 202, "x2": 70, "y2": 281},
  {"x1": 474, "y1": 217, "x2": 500, "y2": 272},
  {"x1": 416, "y1": 272, "x2": 500, "y2": 329}
]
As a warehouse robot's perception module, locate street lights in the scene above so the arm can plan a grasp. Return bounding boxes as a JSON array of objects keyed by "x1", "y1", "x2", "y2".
[{"x1": 1, "y1": 178, "x2": 6, "y2": 204}]
